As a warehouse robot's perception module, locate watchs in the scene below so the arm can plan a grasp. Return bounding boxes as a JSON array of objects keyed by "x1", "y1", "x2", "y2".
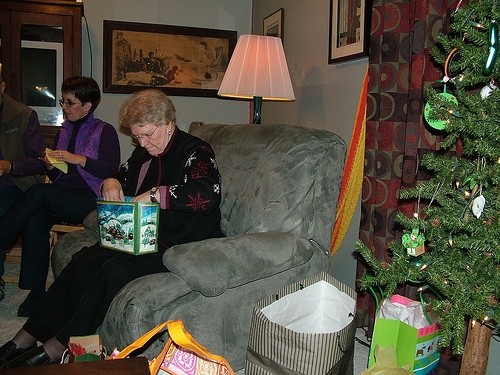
[{"x1": 150, "y1": 187, "x2": 159, "y2": 203}]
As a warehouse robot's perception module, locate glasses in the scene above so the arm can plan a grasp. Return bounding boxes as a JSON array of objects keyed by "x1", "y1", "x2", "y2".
[
  {"x1": 130, "y1": 125, "x2": 160, "y2": 140},
  {"x1": 59, "y1": 99, "x2": 81, "y2": 107}
]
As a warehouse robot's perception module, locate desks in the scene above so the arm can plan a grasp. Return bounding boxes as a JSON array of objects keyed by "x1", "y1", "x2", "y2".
[{"x1": 0, "y1": 357, "x2": 153, "y2": 375}]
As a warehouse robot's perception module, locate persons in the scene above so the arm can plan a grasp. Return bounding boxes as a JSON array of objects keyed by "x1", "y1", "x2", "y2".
[
  {"x1": 0, "y1": 89, "x2": 226, "y2": 372},
  {"x1": 0, "y1": 76, "x2": 121, "y2": 318},
  {"x1": 0, "y1": 77, "x2": 46, "y2": 303}
]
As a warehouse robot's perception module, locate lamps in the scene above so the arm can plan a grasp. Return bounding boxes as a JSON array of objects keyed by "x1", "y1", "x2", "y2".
[{"x1": 217, "y1": 32, "x2": 296, "y2": 124}]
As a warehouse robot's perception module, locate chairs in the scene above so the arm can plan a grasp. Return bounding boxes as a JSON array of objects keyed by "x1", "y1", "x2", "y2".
[{"x1": 39, "y1": 168, "x2": 91, "y2": 291}]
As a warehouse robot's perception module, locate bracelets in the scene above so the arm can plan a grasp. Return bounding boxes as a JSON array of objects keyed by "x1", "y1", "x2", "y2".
[{"x1": 8, "y1": 162, "x2": 12, "y2": 173}]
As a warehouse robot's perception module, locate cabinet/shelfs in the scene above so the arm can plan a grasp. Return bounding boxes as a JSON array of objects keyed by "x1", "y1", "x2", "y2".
[{"x1": 0, "y1": 0, "x2": 85, "y2": 161}]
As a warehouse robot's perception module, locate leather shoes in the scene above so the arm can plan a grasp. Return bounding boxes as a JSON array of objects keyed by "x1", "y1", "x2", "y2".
[
  {"x1": 1, "y1": 344, "x2": 60, "y2": 368},
  {"x1": 0, "y1": 340, "x2": 38, "y2": 367}
]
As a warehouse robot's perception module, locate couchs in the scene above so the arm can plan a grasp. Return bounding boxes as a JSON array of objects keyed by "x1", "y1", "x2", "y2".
[{"x1": 48, "y1": 122, "x2": 346, "y2": 365}]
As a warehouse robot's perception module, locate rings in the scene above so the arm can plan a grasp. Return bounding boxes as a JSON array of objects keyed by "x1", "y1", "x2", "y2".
[{"x1": 59, "y1": 151, "x2": 62, "y2": 155}]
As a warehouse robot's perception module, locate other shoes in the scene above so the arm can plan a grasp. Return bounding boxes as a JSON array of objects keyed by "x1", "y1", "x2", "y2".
[
  {"x1": 17, "y1": 291, "x2": 47, "y2": 317},
  {"x1": 0, "y1": 288, "x2": 5, "y2": 302}
]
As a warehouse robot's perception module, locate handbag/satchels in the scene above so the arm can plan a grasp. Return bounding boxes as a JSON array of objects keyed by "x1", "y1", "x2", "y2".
[
  {"x1": 113, "y1": 319, "x2": 236, "y2": 375},
  {"x1": 365, "y1": 273, "x2": 441, "y2": 375},
  {"x1": 245, "y1": 271, "x2": 358, "y2": 375}
]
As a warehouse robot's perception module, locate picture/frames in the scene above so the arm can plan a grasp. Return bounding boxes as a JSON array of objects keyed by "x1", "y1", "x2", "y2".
[
  {"x1": 103, "y1": 18, "x2": 238, "y2": 100},
  {"x1": 263, "y1": 8, "x2": 285, "y2": 46},
  {"x1": 327, "y1": 0, "x2": 373, "y2": 64}
]
